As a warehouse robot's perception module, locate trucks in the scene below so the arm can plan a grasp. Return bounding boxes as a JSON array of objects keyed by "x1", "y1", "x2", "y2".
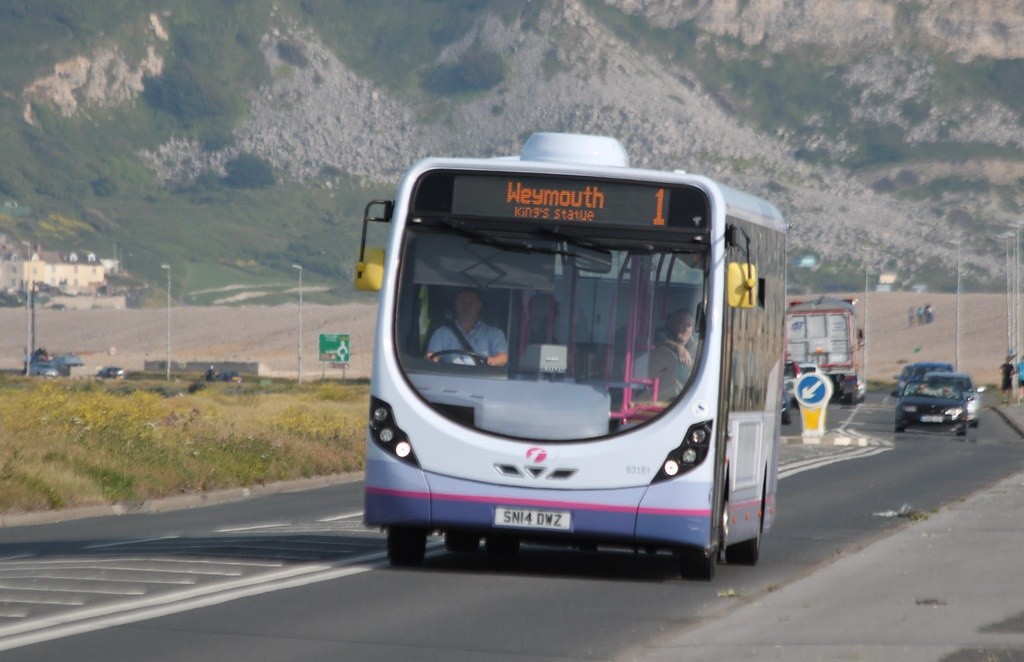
[{"x1": 782, "y1": 296, "x2": 866, "y2": 406}]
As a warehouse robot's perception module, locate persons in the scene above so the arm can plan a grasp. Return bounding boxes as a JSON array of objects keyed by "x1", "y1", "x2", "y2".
[
  {"x1": 653, "y1": 308, "x2": 698, "y2": 387},
  {"x1": 423, "y1": 288, "x2": 508, "y2": 365},
  {"x1": 942, "y1": 387, "x2": 949, "y2": 398},
  {"x1": 205, "y1": 365, "x2": 216, "y2": 382},
  {"x1": 999, "y1": 355, "x2": 1016, "y2": 404},
  {"x1": 23, "y1": 352, "x2": 28, "y2": 370},
  {"x1": 1017, "y1": 356, "x2": 1024, "y2": 402},
  {"x1": 915, "y1": 384, "x2": 925, "y2": 394},
  {"x1": 907, "y1": 304, "x2": 934, "y2": 327}
]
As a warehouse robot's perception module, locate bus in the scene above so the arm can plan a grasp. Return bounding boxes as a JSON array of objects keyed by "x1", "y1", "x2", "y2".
[{"x1": 355, "y1": 131, "x2": 788, "y2": 583}]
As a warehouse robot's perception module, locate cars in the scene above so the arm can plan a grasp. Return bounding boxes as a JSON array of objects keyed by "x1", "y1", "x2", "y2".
[
  {"x1": 780, "y1": 360, "x2": 822, "y2": 427},
  {"x1": 893, "y1": 362, "x2": 987, "y2": 427},
  {"x1": 218, "y1": 370, "x2": 243, "y2": 384},
  {"x1": 100, "y1": 366, "x2": 125, "y2": 381},
  {"x1": 891, "y1": 379, "x2": 973, "y2": 437}
]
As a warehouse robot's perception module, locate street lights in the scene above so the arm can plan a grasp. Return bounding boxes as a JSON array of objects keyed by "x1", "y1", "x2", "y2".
[
  {"x1": 995, "y1": 223, "x2": 1020, "y2": 397},
  {"x1": 21, "y1": 241, "x2": 31, "y2": 377},
  {"x1": 160, "y1": 265, "x2": 171, "y2": 382},
  {"x1": 947, "y1": 239, "x2": 961, "y2": 371},
  {"x1": 292, "y1": 264, "x2": 302, "y2": 385}
]
